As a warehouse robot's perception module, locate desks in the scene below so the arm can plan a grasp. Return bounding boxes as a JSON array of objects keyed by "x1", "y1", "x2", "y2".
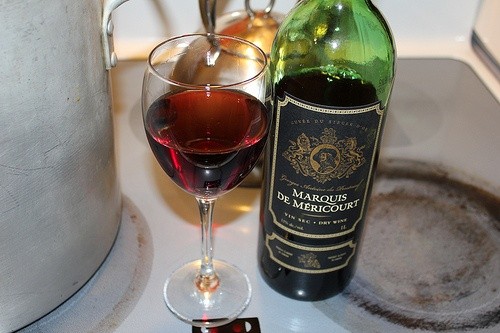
[{"x1": 0, "y1": 55, "x2": 499, "y2": 333}]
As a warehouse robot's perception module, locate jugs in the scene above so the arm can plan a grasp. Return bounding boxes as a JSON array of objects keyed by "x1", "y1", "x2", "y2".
[{"x1": 170, "y1": 0, "x2": 289, "y2": 188}]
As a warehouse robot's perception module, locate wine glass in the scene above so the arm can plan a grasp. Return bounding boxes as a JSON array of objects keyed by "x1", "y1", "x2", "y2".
[{"x1": 141, "y1": 33, "x2": 270, "y2": 328}]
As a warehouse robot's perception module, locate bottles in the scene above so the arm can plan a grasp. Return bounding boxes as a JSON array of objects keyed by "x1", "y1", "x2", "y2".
[{"x1": 257, "y1": 0, "x2": 395, "y2": 302}]
